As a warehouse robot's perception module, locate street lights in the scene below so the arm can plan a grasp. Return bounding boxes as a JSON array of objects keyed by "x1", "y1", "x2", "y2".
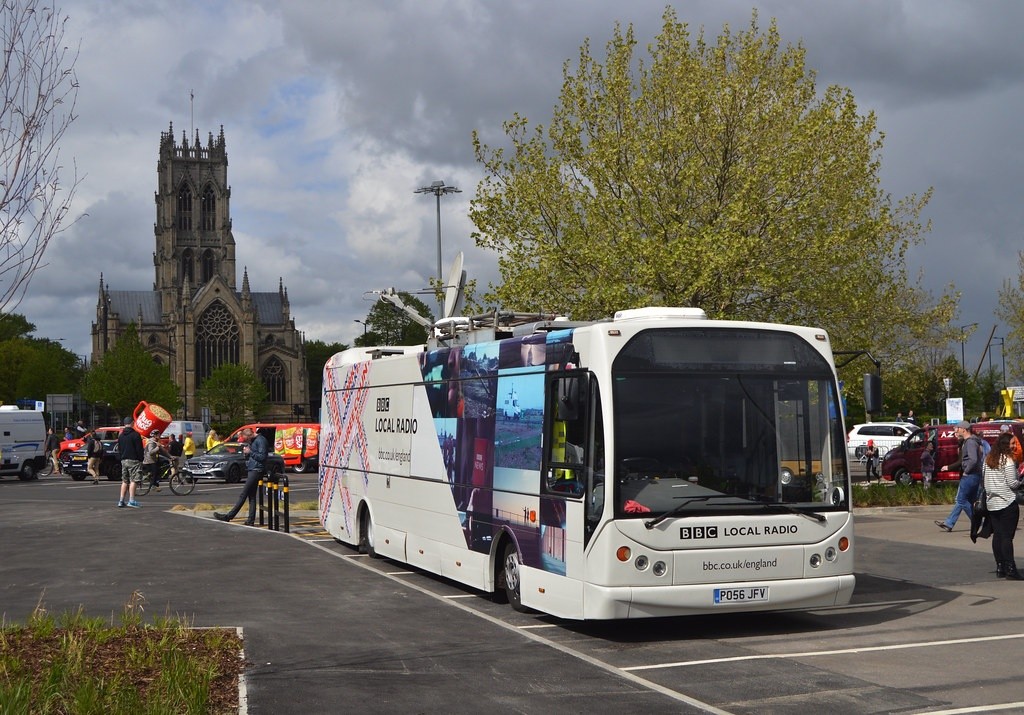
[
  {"x1": 413, "y1": 180, "x2": 462, "y2": 319},
  {"x1": 961, "y1": 322, "x2": 979, "y2": 415},
  {"x1": 989, "y1": 337, "x2": 1005, "y2": 389}
]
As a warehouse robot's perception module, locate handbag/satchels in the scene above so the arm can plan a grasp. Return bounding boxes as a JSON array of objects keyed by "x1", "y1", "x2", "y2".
[
  {"x1": 156, "y1": 461, "x2": 162, "y2": 469},
  {"x1": 973, "y1": 484, "x2": 989, "y2": 517},
  {"x1": 873, "y1": 456, "x2": 879, "y2": 467}
]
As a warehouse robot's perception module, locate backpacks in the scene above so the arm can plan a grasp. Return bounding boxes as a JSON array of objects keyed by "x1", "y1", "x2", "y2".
[
  {"x1": 963, "y1": 436, "x2": 991, "y2": 473},
  {"x1": 91, "y1": 438, "x2": 103, "y2": 453}
]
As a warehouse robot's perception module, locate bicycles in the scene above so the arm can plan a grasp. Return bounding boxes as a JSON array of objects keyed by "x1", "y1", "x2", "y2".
[{"x1": 127, "y1": 457, "x2": 195, "y2": 496}]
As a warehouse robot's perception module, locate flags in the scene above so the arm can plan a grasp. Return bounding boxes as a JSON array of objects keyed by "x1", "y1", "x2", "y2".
[{"x1": 191, "y1": 93, "x2": 194, "y2": 99}]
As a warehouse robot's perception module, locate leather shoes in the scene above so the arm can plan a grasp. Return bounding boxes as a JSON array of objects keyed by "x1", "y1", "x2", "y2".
[{"x1": 934, "y1": 520, "x2": 952, "y2": 532}]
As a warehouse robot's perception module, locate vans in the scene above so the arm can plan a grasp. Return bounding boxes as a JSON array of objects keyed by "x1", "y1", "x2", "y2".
[
  {"x1": 0, "y1": 399, "x2": 47, "y2": 481},
  {"x1": 882, "y1": 423, "x2": 1024, "y2": 490},
  {"x1": 222, "y1": 422, "x2": 320, "y2": 474},
  {"x1": 503, "y1": 399, "x2": 521, "y2": 420}
]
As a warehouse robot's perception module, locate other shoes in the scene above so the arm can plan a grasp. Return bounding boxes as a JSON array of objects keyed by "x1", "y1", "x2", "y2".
[
  {"x1": 214, "y1": 512, "x2": 231, "y2": 522},
  {"x1": 242, "y1": 521, "x2": 254, "y2": 526},
  {"x1": 877, "y1": 478, "x2": 880, "y2": 483},
  {"x1": 150, "y1": 480, "x2": 159, "y2": 487},
  {"x1": 93, "y1": 478, "x2": 99, "y2": 485}
]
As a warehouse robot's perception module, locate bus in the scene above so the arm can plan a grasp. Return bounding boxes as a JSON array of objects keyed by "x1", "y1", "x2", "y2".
[{"x1": 318, "y1": 306, "x2": 856, "y2": 621}]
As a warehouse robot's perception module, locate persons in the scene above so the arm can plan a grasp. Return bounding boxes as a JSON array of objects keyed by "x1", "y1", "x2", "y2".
[
  {"x1": 87, "y1": 433, "x2": 104, "y2": 485},
  {"x1": 237, "y1": 431, "x2": 247, "y2": 443},
  {"x1": 906, "y1": 410, "x2": 917, "y2": 425},
  {"x1": 183, "y1": 432, "x2": 195, "y2": 459},
  {"x1": 118, "y1": 416, "x2": 145, "y2": 509},
  {"x1": 206, "y1": 430, "x2": 221, "y2": 450},
  {"x1": 45, "y1": 427, "x2": 61, "y2": 474},
  {"x1": 143, "y1": 429, "x2": 176, "y2": 487},
  {"x1": 213, "y1": 428, "x2": 268, "y2": 527},
  {"x1": 76, "y1": 420, "x2": 88, "y2": 438},
  {"x1": 896, "y1": 430, "x2": 904, "y2": 436},
  {"x1": 895, "y1": 412, "x2": 904, "y2": 422},
  {"x1": 168, "y1": 433, "x2": 184, "y2": 481},
  {"x1": 63, "y1": 427, "x2": 73, "y2": 441},
  {"x1": 920, "y1": 420, "x2": 1024, "y2": 580},
  {"x1": 865, "y1": 439, "x2": 880, "y2": 483}
]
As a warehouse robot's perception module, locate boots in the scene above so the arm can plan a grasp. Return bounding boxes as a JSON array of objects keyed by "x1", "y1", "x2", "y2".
[
  {"x1": 996, "y1": 561, "x2": 1006, "y2": 577},
  {"x1": 1005, "y1": 561, "x2": 1024, "y2": 580}
]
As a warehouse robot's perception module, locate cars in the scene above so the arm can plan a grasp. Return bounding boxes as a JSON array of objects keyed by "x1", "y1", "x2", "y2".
[
  {"x1": 63, "y1": 441, "x2": 177, "y2": 481},
  {"x1": 178, "y1": 443, "x2": 285, "y2": 485}
]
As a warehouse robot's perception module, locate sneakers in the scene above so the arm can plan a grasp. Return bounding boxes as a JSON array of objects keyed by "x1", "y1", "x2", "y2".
[
  {"x1": 127, "y1": 501, "x2": 142, "y2": 508},
  {"x1": 118, "y1": 501, "x2": 127, "y2": 507}
]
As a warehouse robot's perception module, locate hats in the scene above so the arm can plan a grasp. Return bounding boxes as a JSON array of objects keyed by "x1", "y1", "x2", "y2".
[
  {"x1": 867, "y1": 440, "x2": 873, "y2": 447},
  {"x1": 955, "y1": 420, "x2": 970, "y2": 429},
  {"x1": 150, "y1": 430, "x2": 160, "y2": 438},
  {"x1": 124, "y1": 416, "x2": 133, "y2": 424}
]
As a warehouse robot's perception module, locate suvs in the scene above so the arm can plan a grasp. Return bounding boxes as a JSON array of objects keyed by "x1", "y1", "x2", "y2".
[
  {"x1": 847, "y1": 422, "x2": 936, "y2": 462},
  {"x1": 37, "y1": 421, "x2": 219, "y2": 477}
]
